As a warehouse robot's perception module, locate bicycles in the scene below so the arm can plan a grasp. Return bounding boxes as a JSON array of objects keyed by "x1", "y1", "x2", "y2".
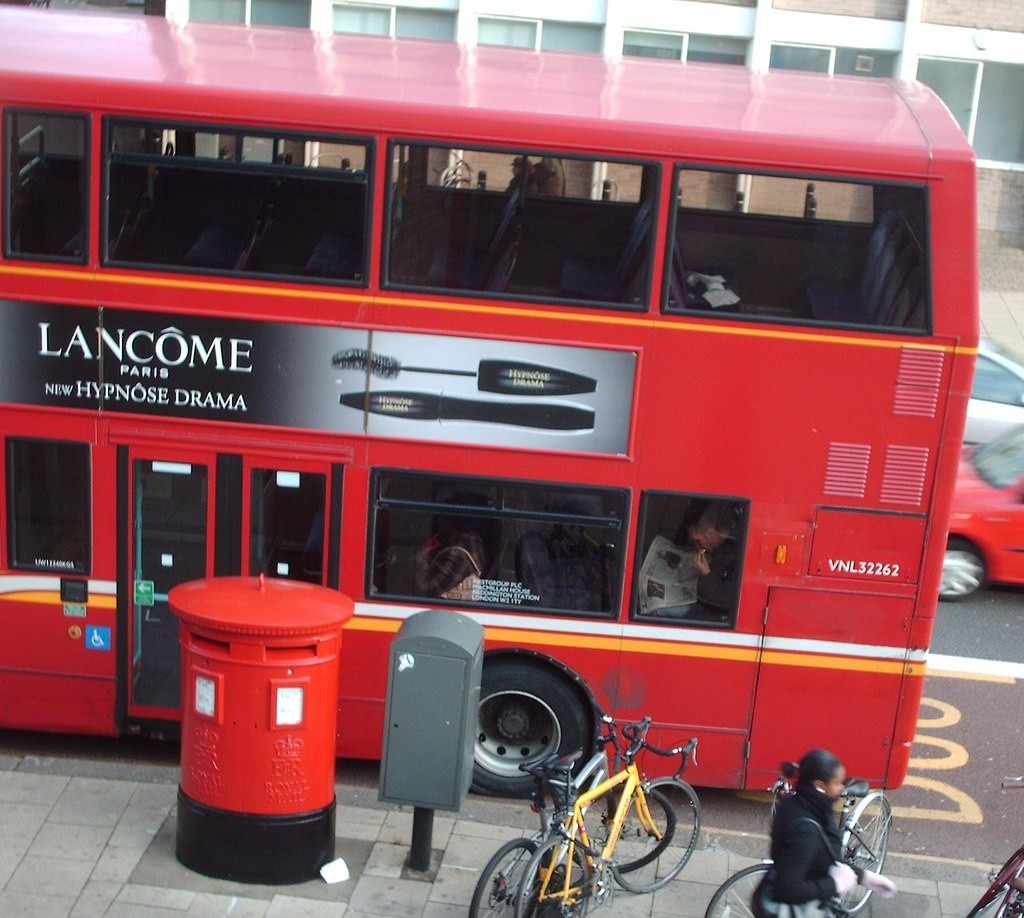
[
  {"x1": 963, "y1": 777, "x2": 1024, "y2": 918},
  {"x1": 468, "y1": 695, "x2": 701, "y2": 917},
  {"x1": 704, "y1": 777, "x2": 894, "y2": 916}
]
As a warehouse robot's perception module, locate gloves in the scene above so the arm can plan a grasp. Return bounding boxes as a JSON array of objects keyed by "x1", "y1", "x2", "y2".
[
  {"x1": 828, "y1": 861, "x2": 859, "y2": 896},
  {"x1": 862, "y1": 870, "x2": 897, "y2": 898}
]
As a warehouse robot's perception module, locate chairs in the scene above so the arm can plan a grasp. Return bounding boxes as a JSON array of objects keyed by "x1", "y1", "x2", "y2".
[
  {"x1": 185, "y1": 172, "x2": 287, "y2": 269},
  {"x1": 306, "y1": 227, "x2": 363, "y2": 279},
  {"x1": 60, "y1": 143, "x2": 175, "y2": 260},
  {"x1": 807, "y1": 210, "x2": 927, "y2": 332},
  {"x1": 450, "y1": 174, "x2": 529, "y2": 292},
  {"x1": 485, "y1": 534, "x2": 510, "y2": 581},
  {"x1": 561, "y1": 192, "x2": 740, "y2": 313}
]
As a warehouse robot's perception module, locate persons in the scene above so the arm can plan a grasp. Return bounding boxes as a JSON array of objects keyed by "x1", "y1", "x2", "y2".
[
  {"x1": 305, "y1": 487, "x2": 739, "y2": 625},
  {"x1": 505, "y1": 156, "x2": 559, "y2": 195},
  {"x1": 769, "y1": 749, "x2": 897, "y2": 918}
]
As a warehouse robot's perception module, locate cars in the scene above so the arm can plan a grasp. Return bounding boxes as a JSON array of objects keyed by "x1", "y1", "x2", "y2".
[
  {"x1": 961, "y1": 332, "x2": 1024, "y2": 450},
  {"x1": 939, "y1": 416, "x2": 1024, "y2": 600}
]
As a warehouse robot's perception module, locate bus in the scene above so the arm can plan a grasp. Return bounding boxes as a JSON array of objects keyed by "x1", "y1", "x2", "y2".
[{"x1": 1, "y1": 2, "x2": 973, "y2": 810}]
